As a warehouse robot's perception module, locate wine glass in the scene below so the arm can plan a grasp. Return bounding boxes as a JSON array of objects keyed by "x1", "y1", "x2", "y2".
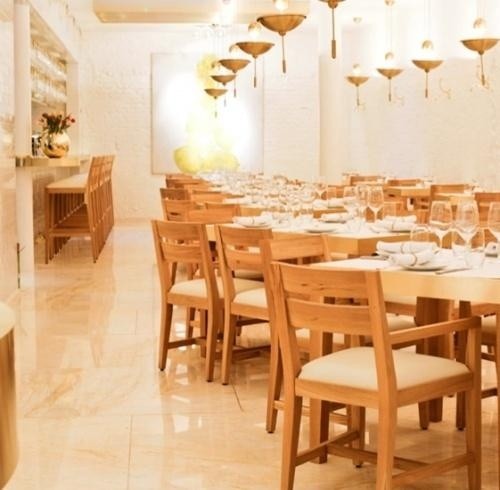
[
  {"x1": 454, "y1": 199, "x2": 480, "y2": 257},
  {"x1": 428, "y1": 201, "x2": 454, "y2": 258},
  {"x1": 487, "y1": 202, "x2": 500, "y2": 266},
  {"x1": 225, "y1": 173, "x2": 386, "y2": 227}
]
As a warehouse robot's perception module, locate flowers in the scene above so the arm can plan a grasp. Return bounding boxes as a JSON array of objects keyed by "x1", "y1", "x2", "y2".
[{"x1": 39, "y1": 113, "x2": 77, "y2": 135}]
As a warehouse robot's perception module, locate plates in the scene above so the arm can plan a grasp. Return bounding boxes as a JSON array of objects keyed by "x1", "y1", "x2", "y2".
[
  {"x1": 398, "y1": 263, "x2": 449, "y2": 270},
  {"x1": 303, "y1": 227, "x2": 335, "y2": 233}
]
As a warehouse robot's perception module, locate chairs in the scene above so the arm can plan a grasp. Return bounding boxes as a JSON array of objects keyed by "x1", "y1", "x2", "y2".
[
  {"x1": 154, "y1": 171, "x2": 498, "y2": 464},
  {"x1": 43, "y1": 156, "x2": 117, "y2": 262},
  {"x1": 271, "y1": 261, "x2": 483, "y2": 489}
]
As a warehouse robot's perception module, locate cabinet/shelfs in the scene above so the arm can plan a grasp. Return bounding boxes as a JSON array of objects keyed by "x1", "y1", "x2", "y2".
[{"x1": 30, "y1": 48, "x2": 68, "y2": 110}]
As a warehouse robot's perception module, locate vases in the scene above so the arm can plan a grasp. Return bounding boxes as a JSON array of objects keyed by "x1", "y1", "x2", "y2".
[{"x1": 41, "y1": 131, "x2": 71, "y2": 158}]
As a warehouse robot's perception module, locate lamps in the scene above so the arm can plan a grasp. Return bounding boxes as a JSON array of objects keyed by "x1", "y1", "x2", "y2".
[{"x1": 202, "y1": 1, "x2": 500, "y2": 118}]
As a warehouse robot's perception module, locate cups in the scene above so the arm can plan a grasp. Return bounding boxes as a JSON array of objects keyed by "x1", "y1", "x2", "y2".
[
  {"x1": 466, "y1": 226, "x2": 485, "y2": 269},
  {"x1": 410, "y1": 225, "x2": 430, "y2": 255},
  {"x1": 381, "y1": 202, "x2": 396, "y2": 221}
]
